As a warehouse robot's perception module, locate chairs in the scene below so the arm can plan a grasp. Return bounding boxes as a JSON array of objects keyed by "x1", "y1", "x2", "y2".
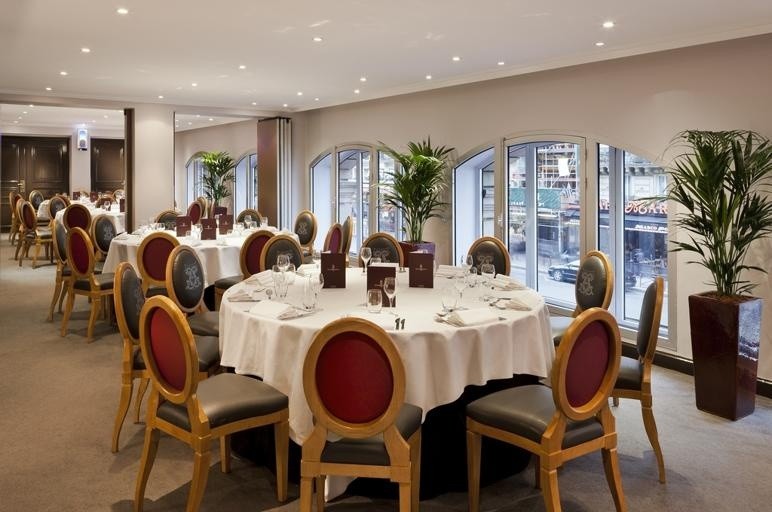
[{"x1": 9, "y1": 187, "x2": 666, "y2": 512}]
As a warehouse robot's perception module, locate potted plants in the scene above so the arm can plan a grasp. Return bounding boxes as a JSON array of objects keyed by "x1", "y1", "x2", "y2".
[
  {"x1": 189, "y1": 149, "x2": 241, "y2": 218},
  {"x1": 377, "y1": 135, "x2": 455, "y2": 266},
  {"x1": 632, "y1": 127, "x2": 772, "y2": 420}
]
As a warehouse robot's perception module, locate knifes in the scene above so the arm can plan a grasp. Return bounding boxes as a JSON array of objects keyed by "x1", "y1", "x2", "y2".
[
  {"x1": 401, "y1": 319, "x2": 406, "y2": 330},
  {"x1": 395, "y1": 317, "x2": 400, "y2": 330}
]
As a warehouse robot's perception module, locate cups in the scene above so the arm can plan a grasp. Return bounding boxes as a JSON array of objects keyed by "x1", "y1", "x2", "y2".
[
  {"x1": 366, "y1": 289, "x2": 383, "y2": 315},
  {"x1": 371, "y1": 258, "x2": 381, "y2": 265}
]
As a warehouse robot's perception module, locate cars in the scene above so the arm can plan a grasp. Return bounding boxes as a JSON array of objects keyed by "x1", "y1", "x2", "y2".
[
  {"x1": 509, "y1": 232, "x2": 527, "y2": 252},
  {"x1": 349, "y1": 186, "x2": 405, "y2": 235},
  {"x1": 545, "y1": 256, "x2": 637, "y2": 291}
]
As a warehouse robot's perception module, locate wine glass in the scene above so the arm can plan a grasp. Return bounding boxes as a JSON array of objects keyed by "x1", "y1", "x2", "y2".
[
  {"x1": 360, "y1": 247, "x2": 372, "y2": 275},
  {"x1": 431, "y1": 253, "x2": 513, "y2": 324},
  {"x1": 86, "y1": 192, "x2": 124, "y2": 215},
  {"x1": 56, "y1": 192, "x2": 86, "y2": 202},
  {"x1": 384, "y1": 277, "x2": 401, "y2": 315},
  {"x1": 135, "y1": 216, "x2": 270, "y2": 248},
  {"x1": 230, "y1": 254, "x2": 328, "y2": 317}
]
